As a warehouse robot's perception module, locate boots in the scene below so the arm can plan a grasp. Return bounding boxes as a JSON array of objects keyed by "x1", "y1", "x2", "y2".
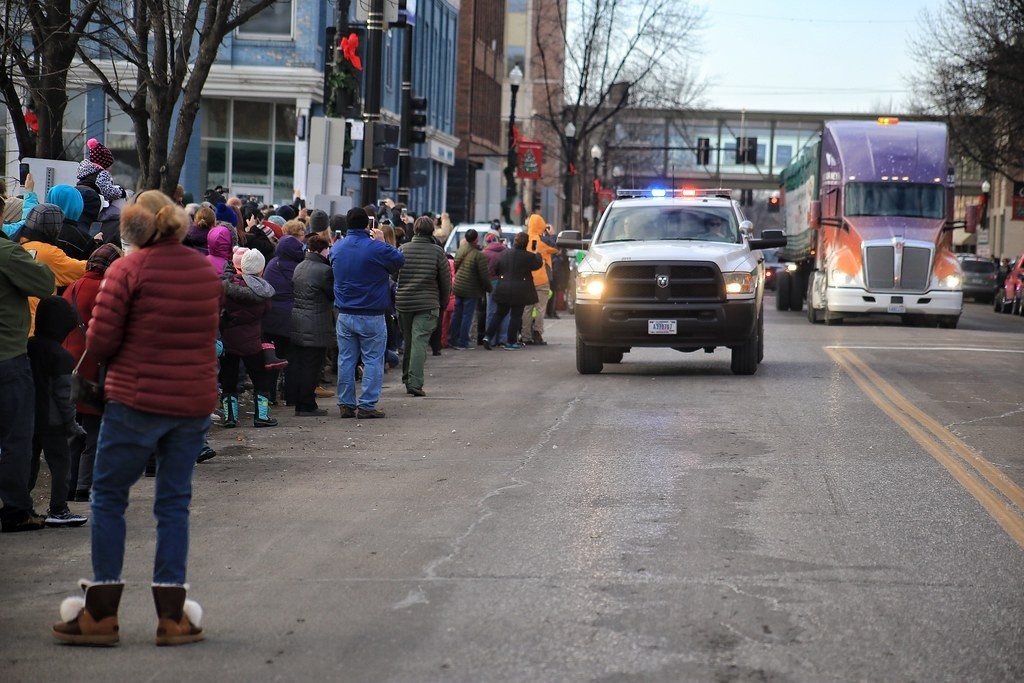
[
  {"x1": 222, "y1": 393, "x2": 239, "y2": 427},
  {"x1": 151, "y1": 583, "x2": 203, "y2": 643},
  {"x1": 51, "y1": 579, "x2": 125, "y2": 644},
  {"x1": 253, "y1": 393, "x2": 278, "y2": 427}
]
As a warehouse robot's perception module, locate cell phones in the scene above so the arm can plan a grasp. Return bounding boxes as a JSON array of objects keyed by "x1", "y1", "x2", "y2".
[
  {"x1": 19, "y1": 163, "x2": 29, "y2": 187},
  {"x1": 89, "y1": 222, "x2": 103, "y2": 236},
  {"x1": 401, "y1": 208, "x2": 407, "y2": 219},
  {"x1": 531, "y1": 240, "x2": 537, "y2": 250},
  {"x1": 368, "y1": 216, "x2": 374, "y2": 232},
  {"x1": 243, "y1": 206, "x2": 252, "y2": 220},
  {"x1": 26, "y1": 249, "x2": 37, "y2": 260},
  {"x1": 300, "y1": 199, "x2": 306, "y2": 210},
  {"x1": 336, "y1": 230, "x2": 341, "y2": 240}
]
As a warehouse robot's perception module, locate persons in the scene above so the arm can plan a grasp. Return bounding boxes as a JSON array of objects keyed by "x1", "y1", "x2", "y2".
[
  {"x1": 990, "y1": 255, "x2": 1011, "y2": 273},
  {"x1": 52, "y1": 191, "x2": 222, "y2": 646},
  {"x1": 616, "y1": 216, "x2": 640, "y2": 238},
  {"x1": 0, "y1": 173, "x2": 571, "y2": 530},
  {"x1": 329, "y1": 207, "x2": 404, "y2": 418},
  {"x1": 390, "y1": 215, "x2": 451, "y2": 395},
  {"x1": 76, "y1": 139, "x2": 127, "y2": 200},
  {"x1": 699, "y1": 215, "x2": 726, "y2": 238}
]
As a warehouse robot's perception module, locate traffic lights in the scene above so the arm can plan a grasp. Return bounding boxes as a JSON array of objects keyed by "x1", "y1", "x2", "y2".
[
  {"x1": 409, "y1": 89, "x2": 426, "y2": 146},
  {"x1": 768, "y1": 197, "x2": 780, "y2": 212}
]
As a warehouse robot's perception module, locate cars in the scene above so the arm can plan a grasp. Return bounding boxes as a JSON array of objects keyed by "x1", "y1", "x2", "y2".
[
  {"x1": 992, "y1": 253, "x2": 1024, "y2": 317},
  {"x1": 951, "y1": 252, "x2": 1000, "y2": 304},
  {"x1": 761, "y1": 247, "x2": 779, "y2": 291}
]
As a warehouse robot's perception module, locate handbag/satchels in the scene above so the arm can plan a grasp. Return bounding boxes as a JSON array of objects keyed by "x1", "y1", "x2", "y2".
[{"x1": 69, "y1": 351, "x2": 106, "y2": 415}]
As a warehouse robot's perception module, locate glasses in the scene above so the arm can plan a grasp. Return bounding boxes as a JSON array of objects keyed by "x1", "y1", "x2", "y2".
[{"x1": 709, "y1": 223, "x2": 721, "y2": 227}]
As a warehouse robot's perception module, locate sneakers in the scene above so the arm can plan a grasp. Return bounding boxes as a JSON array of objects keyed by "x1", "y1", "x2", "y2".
[{"x1": 44, "y1": 512, "x2": 89, "y2": 525}]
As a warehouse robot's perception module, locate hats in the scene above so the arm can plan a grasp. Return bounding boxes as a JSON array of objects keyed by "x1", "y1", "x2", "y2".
[
  {"x1": 347, "y1": 207, "x2": 369, "y2": 230},
  {"x1": 233, "y1": 246, "x2": 251, "y2": 268},
  {"x1": 490, "y1": 219, "x2": 501, "y2": 230},
  {"x1": 215, "y1": 203, "x2": 238, "y2": 227},
  {"x1": 413, "y1": 216, "x2": 434, "y2": 235},
  {"x1": 241, "y1": 248, "x2": 265, "y2": 274},
  {"x1": 485, "y1": 233, "x2": 498, "y2": 245},
  {"x1": 256, "y1": 225, "x2": 274, "y2": 238},
  {"x1": 310, "y1": 209, "x2": 329, "y2": 232},
  {"x1": 87, "y1": 138, "x2": 113, "y2": 168}
]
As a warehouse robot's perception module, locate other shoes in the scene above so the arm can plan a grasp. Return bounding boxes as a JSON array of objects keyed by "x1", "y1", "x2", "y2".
[
  {"x1": 197, "y1": 447, "x2": 216, "y2": 463},
  {"x1": 0, "y1": 513, "x2": 45, "y2": 530},
  {"x1": 145, "y1": 465, "x2": 159, "y2": 477},
  {"x1": 75, "y1": 490, "x2": 90, "y2": 502},
  {"x1": 285, "y1": 330, "x2": 547, "y2": 418}
]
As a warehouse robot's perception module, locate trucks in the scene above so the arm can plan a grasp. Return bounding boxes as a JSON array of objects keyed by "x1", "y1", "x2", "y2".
[{"x1": 777, "y1": 117, "x2": 979, "y2": 330}]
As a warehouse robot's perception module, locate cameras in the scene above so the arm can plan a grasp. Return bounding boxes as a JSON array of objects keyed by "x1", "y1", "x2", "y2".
[
  {"x1": 545, "y1": 226, "x2": 550, "y2": 231},
  {"x1": 378, "y1": 200, "x2": 387, "y2": 207}
]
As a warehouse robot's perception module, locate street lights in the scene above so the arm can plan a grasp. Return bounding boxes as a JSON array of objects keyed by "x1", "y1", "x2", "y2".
[
  {"x1": 562, "y1": 121, "x2": 577, "y2": 229},
  {"x1": 612, "y1": 165, "x2": 622, "y2": 199},
  {"x1": 588, "y1": 142, "x2": 602, "y2": 236},
  {"x1": 980, "y1": 180, "x2": 991, "y2": 231},
  {"x1": 502, "y1": 62, "x2": 523, "y2": 224}
]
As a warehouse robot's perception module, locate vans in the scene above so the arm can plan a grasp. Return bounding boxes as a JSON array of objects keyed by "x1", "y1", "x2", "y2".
[{"x1": 441, "y1": 223, "x2": 527, "y2": 261}]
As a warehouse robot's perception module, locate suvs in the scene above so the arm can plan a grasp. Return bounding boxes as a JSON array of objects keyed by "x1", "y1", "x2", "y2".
[{"x1": 555, "y1": 187, "x2": 788, "y2": 377}]
